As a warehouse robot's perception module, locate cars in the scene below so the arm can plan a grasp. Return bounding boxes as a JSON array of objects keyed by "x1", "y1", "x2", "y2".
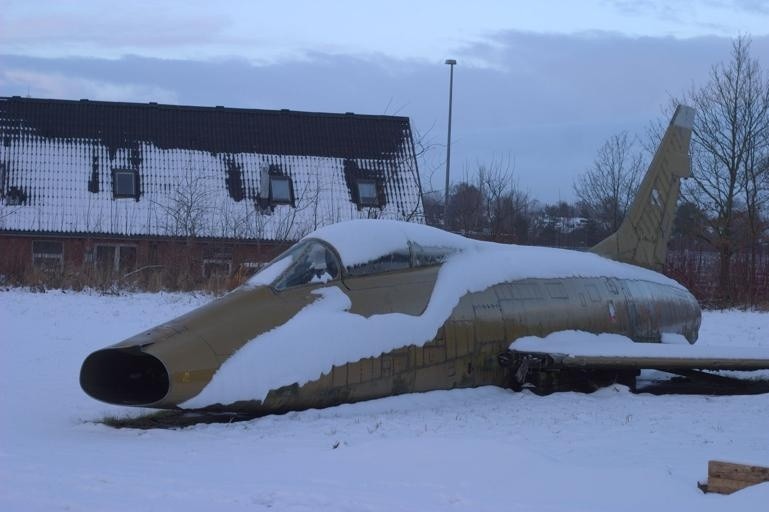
[{"x1": 537, "y1": 213, "x2": 589, "y2": 234}]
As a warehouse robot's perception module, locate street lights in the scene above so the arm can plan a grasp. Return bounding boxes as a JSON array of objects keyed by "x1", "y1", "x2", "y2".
[{"x1": 444, "y1": 59, "x2": 457, "y2": 216}]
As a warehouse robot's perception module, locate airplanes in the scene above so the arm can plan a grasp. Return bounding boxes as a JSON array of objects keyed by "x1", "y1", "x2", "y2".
[{"x1": 79, "y1": 105, "x2": 769, "y2": 415}]
